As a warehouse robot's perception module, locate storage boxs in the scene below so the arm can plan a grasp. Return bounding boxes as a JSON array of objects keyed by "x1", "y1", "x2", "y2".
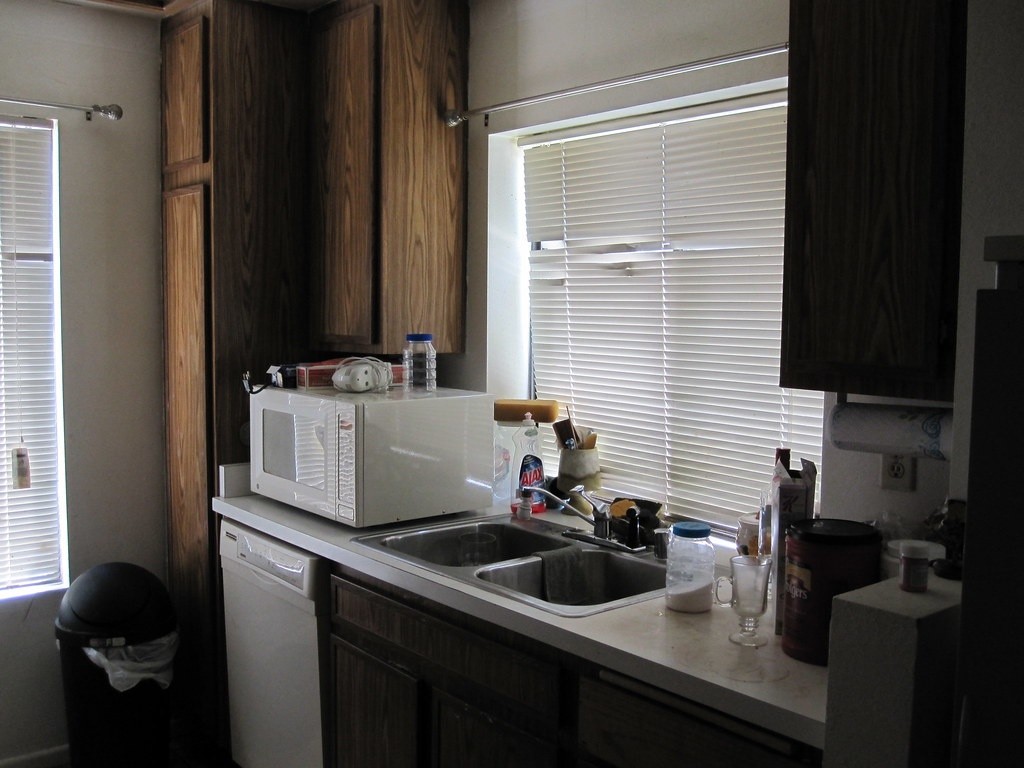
[{"x1": 772, "y1": 448, "x2": 817, "y2": 634}]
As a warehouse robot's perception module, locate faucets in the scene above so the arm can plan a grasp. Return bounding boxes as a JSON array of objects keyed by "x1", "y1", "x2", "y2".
[{"x1": 519, "y1": 484, "x2": 612, "y2": 540}]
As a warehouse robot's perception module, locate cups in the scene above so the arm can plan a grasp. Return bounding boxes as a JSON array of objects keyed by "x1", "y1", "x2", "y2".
[{"x1": 557, "y1": 448, "x2": 601, "y2": 494}]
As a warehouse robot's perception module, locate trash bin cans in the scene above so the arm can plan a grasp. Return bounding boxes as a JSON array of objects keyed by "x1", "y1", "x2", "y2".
[{"x1": 52, "y1": 562, "x2": 183, "y2": 768}]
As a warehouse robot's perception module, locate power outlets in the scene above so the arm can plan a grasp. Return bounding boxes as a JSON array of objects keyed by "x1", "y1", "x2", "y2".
[{"x1": 881, "y1": 454, "x2": 916, "y2": 493}]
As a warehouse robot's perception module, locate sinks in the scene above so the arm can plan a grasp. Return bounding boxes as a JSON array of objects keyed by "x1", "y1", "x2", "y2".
[
  {"x1": 350, "y1": 512, "x2": 589, "y2": 579},
  {"x1": 454, "y1": 549, "x2": 713, "y2": 617}
]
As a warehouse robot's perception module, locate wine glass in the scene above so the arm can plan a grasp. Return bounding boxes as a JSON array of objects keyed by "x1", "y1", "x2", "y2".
[{"x1": 729, "y1": 554, "x2": 772, "y2": 647}]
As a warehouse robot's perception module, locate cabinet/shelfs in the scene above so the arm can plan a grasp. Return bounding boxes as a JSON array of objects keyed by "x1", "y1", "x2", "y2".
[
  {"x1": 311, "y1": 0, "x2": 470, "y2": 356},
  {"x1": 320, "y1": 558, "x2": 572, "y2": 768},
  {"x1": 576, "y1": 668, "x2": 814, "y2": 768},
  {"x1": 781, "y1": 0, "x2": 967, "y2": 399},
  {"x1": 161, "y1": 2, "x2": 311, "y2": 766}
]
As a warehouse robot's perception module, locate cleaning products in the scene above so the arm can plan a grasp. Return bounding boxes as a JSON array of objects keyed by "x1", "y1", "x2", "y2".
[{"x1": 510, "y1": 412, "x2": 547, "y2": 513}]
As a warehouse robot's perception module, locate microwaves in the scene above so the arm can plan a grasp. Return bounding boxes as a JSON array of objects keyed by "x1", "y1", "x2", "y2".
[{"x1": 249, "y1": 384, "x2": 494, "y2": 528}]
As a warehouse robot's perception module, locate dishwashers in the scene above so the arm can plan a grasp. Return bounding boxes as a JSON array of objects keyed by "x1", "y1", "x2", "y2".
[{"x1": 219, "y1": 516, "x2": 324, "y2": 767}]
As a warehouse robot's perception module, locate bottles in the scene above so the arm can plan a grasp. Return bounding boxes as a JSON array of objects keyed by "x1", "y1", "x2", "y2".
[
  {"x1": 402, "y1": 333, "x2": 437, "y2": 394},
  {"x1": 881, "y1": 537, "x2": 946, "y2": 586},
  {"x1": 664, "y1": 521, "x2": 715, "y2": 613},
  {"x1": 510, "y1": 411, "x2": 548, "y2": 515},
  {"x1": 897, "y1": 540, "x2": 930, "y2": 593},
  {"x1": 779, "y1": 515, "x2": 883, "y2": 664}
]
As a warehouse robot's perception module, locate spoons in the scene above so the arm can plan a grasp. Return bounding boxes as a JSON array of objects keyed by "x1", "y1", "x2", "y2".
[{"x1": 583, "y1": 433, "x2": 597, "y2": 450}]
